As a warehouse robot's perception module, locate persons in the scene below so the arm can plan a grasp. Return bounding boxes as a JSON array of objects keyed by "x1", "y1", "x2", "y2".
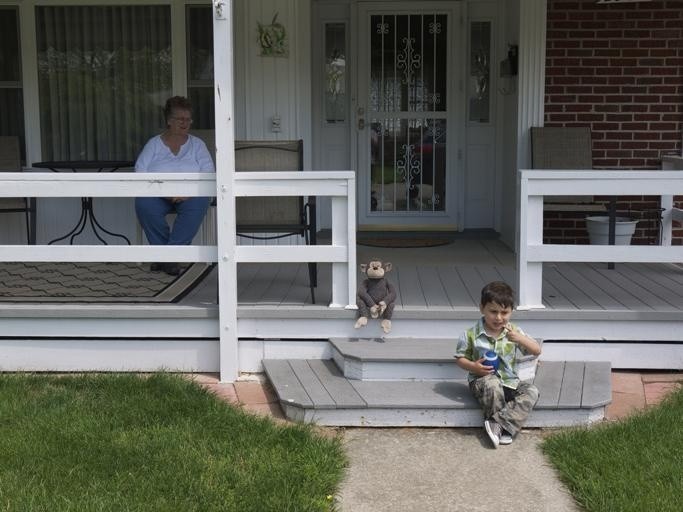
[
  {"x1": 132, "y1": 95, "x2": 214, "y2": 277},
  {"x1": 452, "y1": 281, "x2": 541, "y2": 449}
]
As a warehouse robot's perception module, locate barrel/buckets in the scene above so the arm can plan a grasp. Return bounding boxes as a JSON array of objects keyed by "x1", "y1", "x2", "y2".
[{"x1": 584, "y1": 215, "x2": 639, "y2": 244}]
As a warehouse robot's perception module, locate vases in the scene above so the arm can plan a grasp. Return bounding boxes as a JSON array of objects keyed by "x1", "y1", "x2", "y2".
[{"x1": 583, "y1": 215, "x2": 640, "y2": 245}]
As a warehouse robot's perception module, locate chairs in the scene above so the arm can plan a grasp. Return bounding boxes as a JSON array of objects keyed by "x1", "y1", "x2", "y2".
[
  {"x1": 0, "y1": 135, "x2": 36, "y2": 244},
  {"x1": 211, "y1": 138, "x2": 317, "y2": 289},
  {"x1": 528, "y1": 125, "x2": 616, "y2": 271}
]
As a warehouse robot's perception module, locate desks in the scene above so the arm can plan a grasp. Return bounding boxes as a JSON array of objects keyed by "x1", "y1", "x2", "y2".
[{"x1": 31, "y1": 160, "x2": 136, "y2": 244}]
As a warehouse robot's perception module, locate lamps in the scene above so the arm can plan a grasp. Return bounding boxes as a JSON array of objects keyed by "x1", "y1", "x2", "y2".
[{"x1": 498, "y1": 41, "x2": 519, "y2": 96}]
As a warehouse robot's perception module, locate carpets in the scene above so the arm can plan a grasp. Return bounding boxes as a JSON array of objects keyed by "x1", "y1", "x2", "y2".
[
  {"x1": 356, "y1": 237, "x2": 455, "y2": 248},
  {"x1": 0, "y1": 262, "x2": 216, "y2": 304}
]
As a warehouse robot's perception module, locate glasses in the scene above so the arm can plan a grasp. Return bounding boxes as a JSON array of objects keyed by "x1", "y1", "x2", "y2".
[{"x1": 168, "y1": 115, "x2": 194, "y2": 125}]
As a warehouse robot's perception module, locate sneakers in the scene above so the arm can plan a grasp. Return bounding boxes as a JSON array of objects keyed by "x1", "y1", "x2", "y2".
[
  {"x1": 150, "y1": 262, "x2": 181, "y2": 276},
  {"x1": 483, "y1": 417, "x2": 502, "y2": 450},
  {"x1": 499, "y1": 429, "x2": 513, "y2": 446}
]
